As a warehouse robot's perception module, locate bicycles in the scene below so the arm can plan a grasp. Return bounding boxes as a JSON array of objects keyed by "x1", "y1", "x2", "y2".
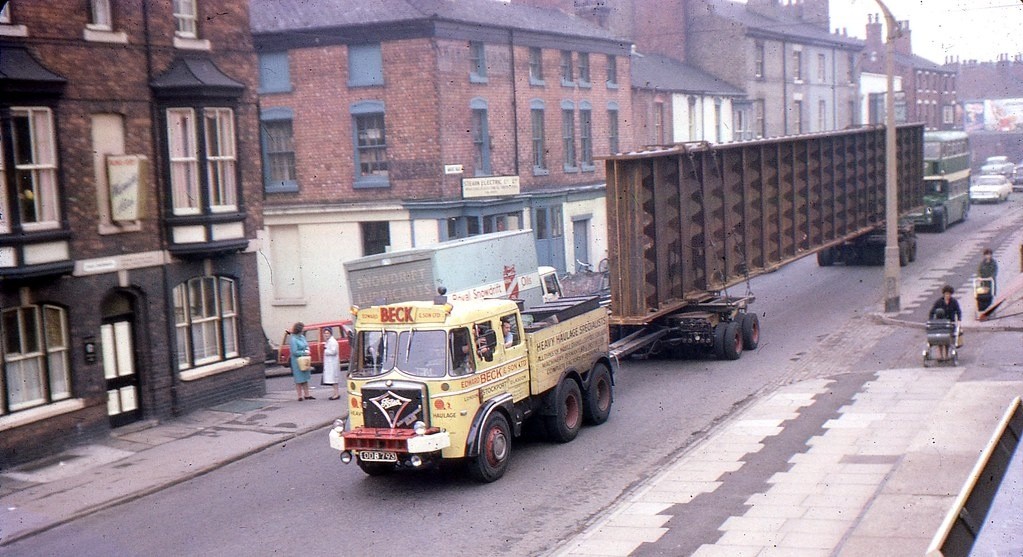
[
  {"x1": 576, "y1": 259, "x2": 594, "y2": 277},
  {"x1": 598, "y1": 248, "x2": 611, "y2": 274}
]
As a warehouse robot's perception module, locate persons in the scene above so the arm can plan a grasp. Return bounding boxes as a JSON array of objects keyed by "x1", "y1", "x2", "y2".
[
  {"x1": 925, "y1": 248, "x2": 997, "y2": 363},
  {"x1": 322, "y1": 326, "x2": 342, "y2": 400},
  {"x1": 290, "y1": 322, "x2": 316, "y2": 401},
  {"x1": 455, "y1": 318, "x2": 514, "y2": 375}
]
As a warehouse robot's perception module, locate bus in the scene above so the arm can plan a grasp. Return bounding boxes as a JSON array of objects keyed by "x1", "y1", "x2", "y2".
[{"x1": 901, "y1": 131, "x2": 973, "y2": 234}]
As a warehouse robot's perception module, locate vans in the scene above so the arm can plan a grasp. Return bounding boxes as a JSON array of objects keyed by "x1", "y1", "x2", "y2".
[{"x1": 276, "y1": 319, "x2": 356, "y2": 371}]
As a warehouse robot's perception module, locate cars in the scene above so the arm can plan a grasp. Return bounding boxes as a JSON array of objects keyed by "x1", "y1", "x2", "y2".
[
  {"x1": 981, "y1": 154, "x2": 1023, "y2": 192},
  {"x1": 968, "y1": 174, "x2": 1012, "y2": 202}
]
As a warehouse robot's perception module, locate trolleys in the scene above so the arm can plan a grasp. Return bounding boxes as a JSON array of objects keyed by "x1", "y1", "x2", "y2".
[
  {"x1": 922, "y1": 318, "x2": 963, "y2": 366},
  {"x1": 974, "y1": 277, "x2": 995, "y2": 320}
]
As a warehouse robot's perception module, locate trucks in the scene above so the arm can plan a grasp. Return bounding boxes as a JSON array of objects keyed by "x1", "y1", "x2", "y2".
[{"x1": 342, "y1": 229, "x2": 564, "y2": 364}]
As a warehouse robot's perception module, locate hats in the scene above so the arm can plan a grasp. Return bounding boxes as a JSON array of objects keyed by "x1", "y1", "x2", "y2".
[{"x1": 323, "y1": 327, "x2": 333, "y2": 334}]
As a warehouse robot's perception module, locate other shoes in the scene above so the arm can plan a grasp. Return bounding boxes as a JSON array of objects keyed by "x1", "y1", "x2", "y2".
[
  {"x1": 298, "y1": 397, "x2": 303, "y2": 402},
  {"x1": 329, "y1": 395, "x2": 340, "y2": 400},
  {"x1": 305, "y1": 396, "x2": 315, "y2": 399},
  {"x1": 937, "y1": 358, "x2": 949, "y2": 363}
]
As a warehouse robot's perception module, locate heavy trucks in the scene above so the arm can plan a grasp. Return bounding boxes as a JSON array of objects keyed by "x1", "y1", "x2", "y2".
[{"x1": 328, "y1": 121, "x2": 931, "y2": 485}]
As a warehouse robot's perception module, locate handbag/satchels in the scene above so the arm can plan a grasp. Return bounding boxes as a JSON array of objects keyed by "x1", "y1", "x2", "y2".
[
  {"x1": 297, "y1": 351, "x2": 312, "y2": 371},
  {"x1": 321, "y1": 376, "x2": 333, "y2": 386}
]
